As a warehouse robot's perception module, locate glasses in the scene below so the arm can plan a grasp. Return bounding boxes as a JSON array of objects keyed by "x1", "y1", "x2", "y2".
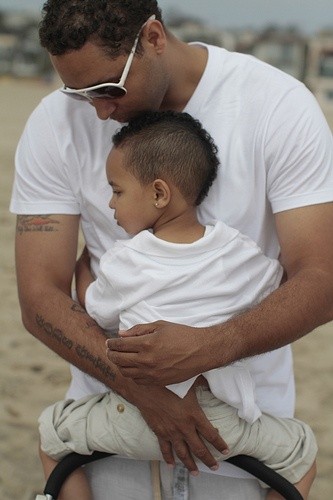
[{"x1": 59, "y1": 14, "x2": 155, "y2": 102}]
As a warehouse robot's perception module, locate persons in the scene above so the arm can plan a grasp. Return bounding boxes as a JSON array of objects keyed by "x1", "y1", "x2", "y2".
[
  {"x1": 10, "y1": 0, "x2": 333, "y2": 500},
  {"x1": 39, "y1": 111, "x2": 318, "y2": 500}
]
